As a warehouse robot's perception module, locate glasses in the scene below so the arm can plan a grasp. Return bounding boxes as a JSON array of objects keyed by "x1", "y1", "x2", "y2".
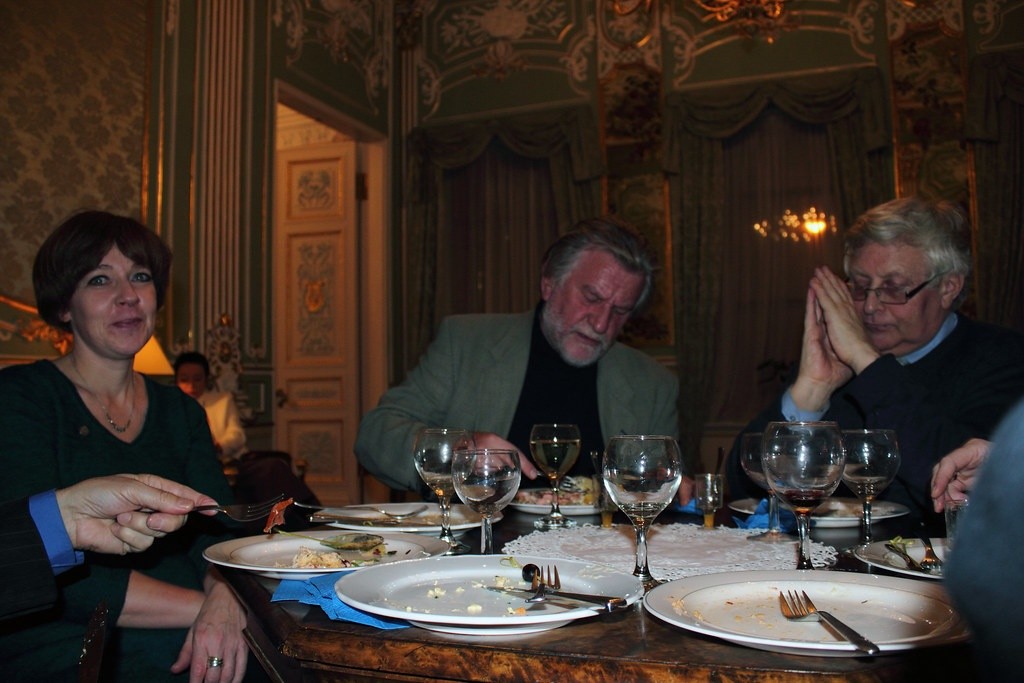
[{"x1": 843, "y1": 273, "x2": 939, "y2": 306}]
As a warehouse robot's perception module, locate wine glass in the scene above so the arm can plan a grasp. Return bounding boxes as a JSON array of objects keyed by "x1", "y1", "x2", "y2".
[
  {"x1": 695, "y1": 474, "x2": 724, "y2": 529},
  {"x1": 413, "y1": 428, "x2": 477, "y2": 556},
  {"x1": 603, "y1": 435, "x2": 683, "y2": 599},
  {"x1": 760, "y1": 420, "x2": 848, "y2": 570},
  {"x1": 451, "y1": 449, "x2": 522, "y2": 555},
  {"x1": 836, "y1": 429, "x2": 901, "y2": 557},
  {"x1": 529, "y1": 424, "x2": 581, "y2": 527},
  {"x1": 740, "y1": 433, "x2": 807, "y2": 543},
  {"x1": 592, "y1": 474, "x2": 623, "y2": 528}
]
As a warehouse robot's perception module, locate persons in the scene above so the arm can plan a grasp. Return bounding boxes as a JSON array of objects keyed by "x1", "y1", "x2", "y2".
[
  {"x1": 0, "y1": 209, "x2": 326, "y2": 683},
  {"x1": 354, "y1": 216, "x2": 697, "y2": 506},
  {"x1": 930, "y1": 399, "x2": 1024, "y2": 683},
  {"x1": 722, "y1": 196, "x2": 1024, "y2": 514}
]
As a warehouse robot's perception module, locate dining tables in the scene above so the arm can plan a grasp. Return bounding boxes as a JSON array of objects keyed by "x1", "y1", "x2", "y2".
[{"x1": 213, "y1": 500, "x2": 994, "y2": 683}]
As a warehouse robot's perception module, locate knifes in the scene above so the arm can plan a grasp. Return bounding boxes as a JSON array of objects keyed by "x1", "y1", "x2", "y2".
[
  {"x1": 483, "y1": 586, "x2": 628, "y2": 609},
  {"x1": 886, "y1": 544, "x2": 931, "y2": 571}
]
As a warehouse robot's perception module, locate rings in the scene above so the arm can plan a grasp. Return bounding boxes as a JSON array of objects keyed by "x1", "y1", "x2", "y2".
[
  {"x1": 954, "y1": 472, "x2": 958, "y2": 481},
  {"x1": 207, "y1": 656, "x2": 224, "y2": 666}
]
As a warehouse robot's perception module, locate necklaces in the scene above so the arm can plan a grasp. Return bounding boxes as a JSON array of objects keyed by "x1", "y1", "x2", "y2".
[
  {"x1": 200, "y1": 390, "x2": 208, "y2": 407},
  {"x1": 72, "y1": 350, "x2": 136, "y2": 432}
]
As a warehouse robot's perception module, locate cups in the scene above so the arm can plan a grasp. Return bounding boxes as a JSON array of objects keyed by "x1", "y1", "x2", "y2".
[{"x1": 944, "y1": 499, "x2": 969, "y2": 554}]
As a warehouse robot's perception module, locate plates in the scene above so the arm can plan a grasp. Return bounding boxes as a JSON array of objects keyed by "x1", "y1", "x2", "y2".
[
  {"x1": 642, "y1": 571, "x2": 977, "y2": 657},
  {"x1": 727, "y1": 496, "x2": 910, "y2": 528},
  {"x1": 853, "y1": 538, "x2": 953, "y2": 579},
  {"x1": 201, "y1": 531, "x2": 645, "y2": 636},
  {"x1": 311, "y1": 503, "x2": 504, "y2": 538},
  {"x1": 506, "y1": 489, "x2": 602, "y2": 515}
]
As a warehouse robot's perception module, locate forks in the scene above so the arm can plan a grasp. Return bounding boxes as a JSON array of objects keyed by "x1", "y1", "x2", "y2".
[
  {"x1": 294, "y1": 502, "x2": 429, "y2": 520},
  {"x1": 524, "y1": 564, "x2": 561, "y2": 604},
  {"x1": 113, "y1": 493, "x2": 285, "y2": 521},
  {"x1": 914, "y1": 520, "x2": 944, "y2": 568},
  {"x1": 536, "y1": 471, "x2": 586, "y2": 493},
  {"x1": 780, "y1": 590, "x2": 880, "y2": 655}
]
